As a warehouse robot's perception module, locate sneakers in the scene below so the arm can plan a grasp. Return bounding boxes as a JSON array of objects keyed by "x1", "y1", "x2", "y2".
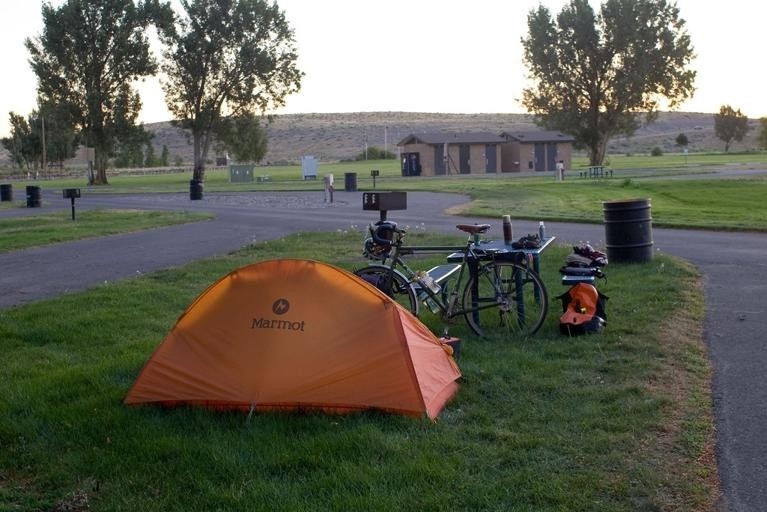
[{"x1": 512, "y1": 233, "x2": 540, "y2": 249}]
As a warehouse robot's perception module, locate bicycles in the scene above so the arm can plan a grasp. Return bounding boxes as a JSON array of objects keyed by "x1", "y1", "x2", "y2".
[{"x1": 352, "y1": 221, "x2": 548, "y2": 341}]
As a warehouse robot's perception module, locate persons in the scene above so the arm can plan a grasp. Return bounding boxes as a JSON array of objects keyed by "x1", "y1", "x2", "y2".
[{"x1": 555, "y1": 159, "x2": 567, "y2": 181}]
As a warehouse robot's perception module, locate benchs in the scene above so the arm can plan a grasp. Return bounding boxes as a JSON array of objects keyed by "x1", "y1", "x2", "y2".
[
  {"x1": 398, "y1": 264, "x2": 462, "y2": 320},
  {"x1": 562, "y1": 264, "x2": 600, "y2": 289}
]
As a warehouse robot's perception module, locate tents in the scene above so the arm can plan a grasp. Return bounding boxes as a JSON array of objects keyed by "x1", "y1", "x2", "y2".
[{"x1": 122, "y1": 258, "x2": 462, "y2": 423}]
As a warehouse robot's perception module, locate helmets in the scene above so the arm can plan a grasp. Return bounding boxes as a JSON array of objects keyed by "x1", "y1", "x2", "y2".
[{"x1": 362, "y1": 239, "x2": 393, "y2": 262}]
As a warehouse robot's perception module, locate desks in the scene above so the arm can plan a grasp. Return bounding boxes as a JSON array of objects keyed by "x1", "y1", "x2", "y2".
[{"x1": 448, "y1": 235, "x2": 556, "y2": 334}]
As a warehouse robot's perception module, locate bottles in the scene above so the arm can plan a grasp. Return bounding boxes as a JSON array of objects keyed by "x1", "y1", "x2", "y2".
[
  {"x1": 502, "y1": 214, "x2": 513, "y2": 242},
  {"x1": 472, "y1": 223, "x2": 480, "y2": 247},
  {"x1": 537, "y1": 221, "x2": 546, "y2": 242},
  {"x1": 416, "y1": 271, "x2": 442, "y2": 314}
]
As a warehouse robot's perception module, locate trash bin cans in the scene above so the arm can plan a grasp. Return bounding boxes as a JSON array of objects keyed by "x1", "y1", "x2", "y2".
[
  {"x1": 0, "y1": 184, "x2": 13, "y2": 201},
  {"x1": 600, "y1": 198, "x2": 654, "y2": 268},
  {"x1": 26, "y1": 186, "x2": 42, "y2": 208},
  {"x1": 344, "y1": 173, "x2": 357, "y2": 191}
]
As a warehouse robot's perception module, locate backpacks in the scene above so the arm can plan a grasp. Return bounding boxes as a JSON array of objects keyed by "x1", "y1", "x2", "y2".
[
  {"x1": 557, "y1": 284, "x2": 608, "y2": 336},
  {"x1": 360, "y1": 273, "x2": 394, "y2": 302}
]
